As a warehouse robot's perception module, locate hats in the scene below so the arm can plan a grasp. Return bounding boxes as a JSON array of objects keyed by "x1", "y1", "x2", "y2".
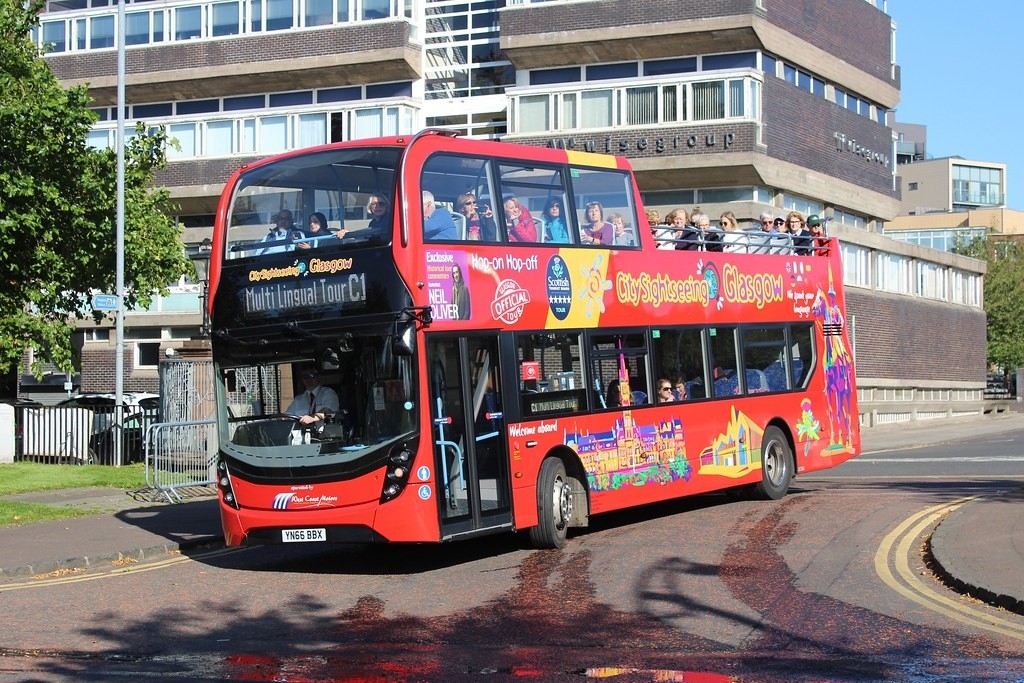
[
  {"x1": 807, "y1": 215, "x2": 820, "y2": 224},
  {"x1": 714, "y1": 367, "x2": 724, "y2": 378}
]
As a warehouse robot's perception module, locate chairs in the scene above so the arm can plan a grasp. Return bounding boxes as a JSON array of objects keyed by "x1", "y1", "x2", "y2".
[
  {"x1": 627, "y1": 360, "x2": 803, "y2": 408},
  {"x1": 327, "y1": 211, "x2": 795, "y2": 258}
]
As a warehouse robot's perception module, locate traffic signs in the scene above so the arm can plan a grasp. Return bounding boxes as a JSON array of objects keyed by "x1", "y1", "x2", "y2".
[{"x1": 90, "y1": 294, "x2": 118, "y2": 311}]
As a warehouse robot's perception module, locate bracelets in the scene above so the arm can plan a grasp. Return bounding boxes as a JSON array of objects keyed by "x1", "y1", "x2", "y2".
[
  {"x1": 340, "y1": 229, "x2": 346, "y2": 235},
  {"x1": 316, "y1": 414, "x2": 323, "y2": 420},
  {"x1": 589, "y1": 237, "x2": 594, "y2": 243}
]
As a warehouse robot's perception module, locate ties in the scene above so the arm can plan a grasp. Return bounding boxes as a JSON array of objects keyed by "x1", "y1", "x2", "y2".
[{"x1": 309, "y1": 392, "x2": 317, "y2": 415}]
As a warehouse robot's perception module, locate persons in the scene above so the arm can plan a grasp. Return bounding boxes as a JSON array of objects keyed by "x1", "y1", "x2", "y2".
[
  {"x1": 759, "y1": 209, "x2": 829, "y2": 256},
  {"x1": 456, "y1": 194, "x2": 496, "y2": 241},
  {"x1": 714, "y1": 366, "x2": 728, "y2": 383},
  {"x1": 502, "y1": 196, "x2": 537, "y2": 243},
  {"x1": 607, "y1": 380, "x2": 632, "y2": 409},
  {"x1": 288, "y1": 212, "x2": 339, "y2": 249},
  {"x1": 450, "y1": 263, "x2": 470, "y2": 320},
  {"x1": 336, "y1": 192, "x2": 390, "y2": 244},
  {"x1": 580, "y1": 201, "x2": 613, "y2": 246},
  {"x1": 541, "y1": 196, "x2": 570, "y2": 244},
  {"x1": 422, "y1": 190, "x2": 460, "y2": 239},
  {"x1": 607, "y1": 212, "x2": 634, "y2": 246},
  {"x1": 254, "y1": 209, "x2": 302, "y2": 256},
  {"x1": 646, "y1": 206, "x2": 750, "y2": 253},
  {"x1": 277, "y1": 363, "x2": 340, "y2": 438},
  {"x1": 658, "y1": 376, "x2": 689, "y2": 405}
]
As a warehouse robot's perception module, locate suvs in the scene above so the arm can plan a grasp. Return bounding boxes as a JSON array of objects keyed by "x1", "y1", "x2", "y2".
[
  {"x1": 56, "y1": 391, "x2": 161, "y2": 467},
  {"x1": 0, "y1": 398, "x2": 45, "y2": 462}
]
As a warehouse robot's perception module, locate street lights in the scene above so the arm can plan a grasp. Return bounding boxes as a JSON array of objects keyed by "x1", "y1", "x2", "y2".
[{"x1": 189, "y1": 238, "x2": 213, "y2": 341}]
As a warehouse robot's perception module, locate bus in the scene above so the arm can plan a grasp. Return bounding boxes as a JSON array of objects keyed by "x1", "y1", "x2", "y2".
[{"x1": 205, "y1": 126, "x2": 863, "y2": 549}]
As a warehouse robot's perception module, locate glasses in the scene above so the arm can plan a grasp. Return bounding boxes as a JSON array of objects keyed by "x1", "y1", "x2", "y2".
[
  {"x1": 719, "y1": 222, "x2": 727, "y2": 226},
  {"x1": 550, "y1": 204, "x2": 560, "y2": 208},
  {"x1": 587, "y1": 201, "x2": 599, "y2": 206},
  {"x1": 300, "y1": 373, "x2": 318, "y2": 380},
  {"x1": 662, "y1": 387, "x2": 673, "y2": 392},
  {"x1": 812, "y1": 224, "x2": 820, "y2": 227},
  {"x1": 277, "y1": 216, "x2": 291, "y2": 222},
  {"x1": 775, "y1": 222, "x2": 783, "y2": 226},
  {"x1": 371, "y1": 202, "x2": 384, "y2": 207},
  {"x1": 763, "y1": 221, "x2": 772, "y2": 224},
  {"x1": 465, "y1": 201, "x2": 477, "y2": 205}
]
